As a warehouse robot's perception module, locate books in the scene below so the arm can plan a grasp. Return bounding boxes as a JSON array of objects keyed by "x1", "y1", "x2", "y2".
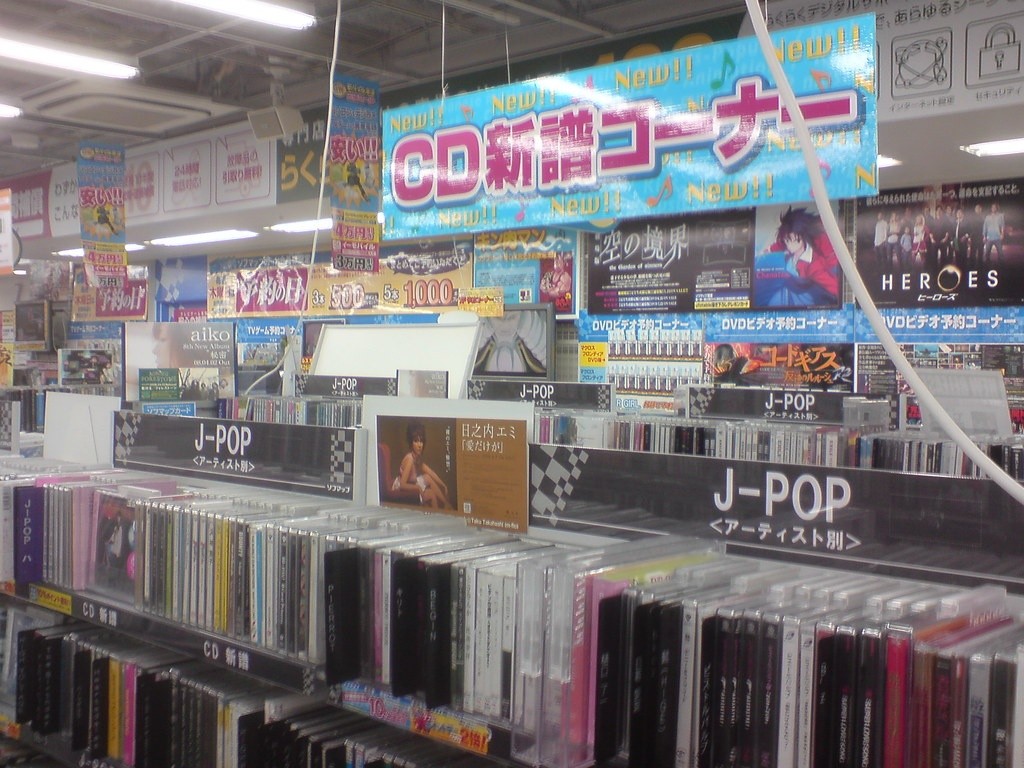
[{"x1": 0, "y1": 395, "x2": 1024, "y2": 768}]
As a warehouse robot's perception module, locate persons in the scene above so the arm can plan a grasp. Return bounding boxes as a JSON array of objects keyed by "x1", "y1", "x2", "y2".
[
  {"x1": 873, "y1": 202, "x2": 1007, "y2": 277},
  {"x1": 391, "y1": 422, "x2": 449, "y2": 513}
]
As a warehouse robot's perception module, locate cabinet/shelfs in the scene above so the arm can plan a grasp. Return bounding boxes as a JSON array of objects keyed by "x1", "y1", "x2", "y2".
[{"x1": 0, "y1": 179, "x2": 1024, "y2": 768}]
